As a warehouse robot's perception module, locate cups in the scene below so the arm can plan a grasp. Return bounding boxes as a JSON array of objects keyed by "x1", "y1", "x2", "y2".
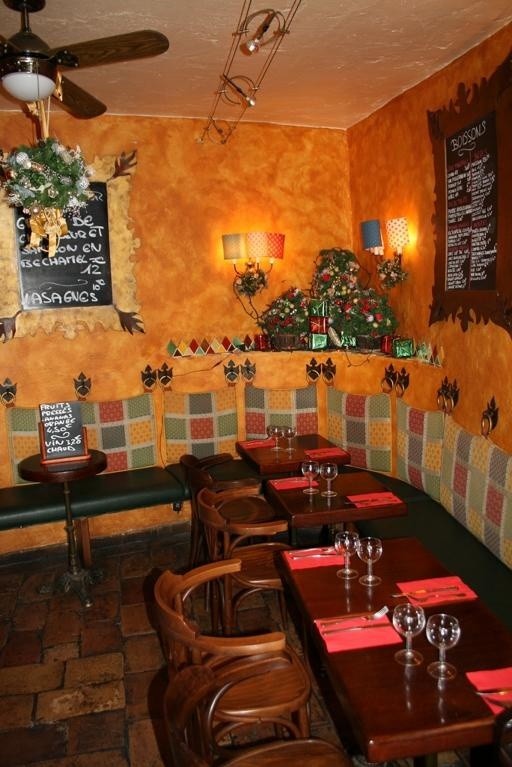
[
  {"x1": 254, "y1": 334, "x2": 267, "y2": 349},
  {"x1": 380, "y1": 335, "x2": 390, "y2": 352}
]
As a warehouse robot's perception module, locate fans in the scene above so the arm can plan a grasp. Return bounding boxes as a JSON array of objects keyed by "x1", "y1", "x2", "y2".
[{"x1": 0, "y1": 0, "x2": 169, "y2": 121}]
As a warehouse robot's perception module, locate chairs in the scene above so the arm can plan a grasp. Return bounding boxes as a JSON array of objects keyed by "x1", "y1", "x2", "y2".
[
  {"x1": 154, "y1": 555, "x2": 313, "y2": 740},
  {"x1": 191, "y1": 487, "x2": 302, "y2": 633},
  {"x1": 156, "y1": 663, "x2": 354, "y2": 767},
  {"x1": 175, "y1": 449, "x2": 262, "y2": 566}
]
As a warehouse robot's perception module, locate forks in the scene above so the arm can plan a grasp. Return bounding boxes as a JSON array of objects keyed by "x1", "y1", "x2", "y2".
[
  {"x1": 485, "y1": 699, "x2": 511, "y2": 708},
  {"x1": 288, "y1": 538, "x2": 344, "y2": 555},
  {"x1": 319, "y1": 605, "x2": 389, "y2": 628},
  {"x1": 406, "y1": 592, "x2": 469, "y2": 601}
]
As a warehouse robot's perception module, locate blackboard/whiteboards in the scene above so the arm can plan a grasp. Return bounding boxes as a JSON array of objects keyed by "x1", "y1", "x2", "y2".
[{"x1": 38, "y1": 401, "x2": 93, "y2": 464}]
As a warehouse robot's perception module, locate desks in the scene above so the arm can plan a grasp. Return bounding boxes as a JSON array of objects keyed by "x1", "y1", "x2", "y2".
[{"x1": 17, "y1": 448, "x2": 109, "y2": 607}]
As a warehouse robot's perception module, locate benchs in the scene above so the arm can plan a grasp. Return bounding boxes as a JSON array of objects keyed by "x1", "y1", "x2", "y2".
[
  {"x1": 165, "y1": 456, "x2": 511, "y2": 637},
  {"x1": 0, "y1": 463, "x2": 184, "y2": 571}
]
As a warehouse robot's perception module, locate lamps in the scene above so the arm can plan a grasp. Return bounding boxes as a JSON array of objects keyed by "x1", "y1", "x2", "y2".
[
  {"x1": 198, "y1": 0, "x2": 302, "y2": 145},
  {"x1": 1, "y1": 52, "x2": 60, "y2": 101},
  {"x1": 221, "y1": 230, "x2": 284, "y2": 293},
  {"x1": 357, "y1": 215, "x2": 410, "y2": 271}
]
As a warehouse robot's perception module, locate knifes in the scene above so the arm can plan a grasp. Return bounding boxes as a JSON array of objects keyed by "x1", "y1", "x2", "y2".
[
  {"x1": 290, "y1": 553, "x2": 340, "y2": 560},
  {"x1": 388, "y1": 583, "x2": 457, "y2": 598},
  {"x1": 467, "y1": 687, "x2": 511, "y2": 697},
  {"x1": 323, "y1": 622, "x2": 387, "y2": 636},
  {"x1": 344, "y1": 497, "x2": 390, "y2": 504}
]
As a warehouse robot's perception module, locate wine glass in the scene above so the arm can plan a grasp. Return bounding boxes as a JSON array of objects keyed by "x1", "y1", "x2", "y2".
[
  {"x1": 426, "y1": 614, "x2": 461, "y2": 680},
  {"x1": 391, "y1": 602, "x2": 424, "y2": 667},
  {"x1": 320, "y1": 462, "x2": 338, "y2": 497},
  {"x1": 268, "y1": 424, "x2": 298, "y2": 454},
  {"x1": 429, "y1": 679, "x2": 456, "y2": 724},
  {"x1": 301, "y1": 460, "x2": 320, "y2": 494},
  {"x1": 355, "y1": 537, "x2": 384, "y2": 587},
  {"x1": 334, "y1": 531, "x2": 360, "y2": 578},
  {"x1": 398, "y1": 664, "x2": 419, "y2": 713}
]
